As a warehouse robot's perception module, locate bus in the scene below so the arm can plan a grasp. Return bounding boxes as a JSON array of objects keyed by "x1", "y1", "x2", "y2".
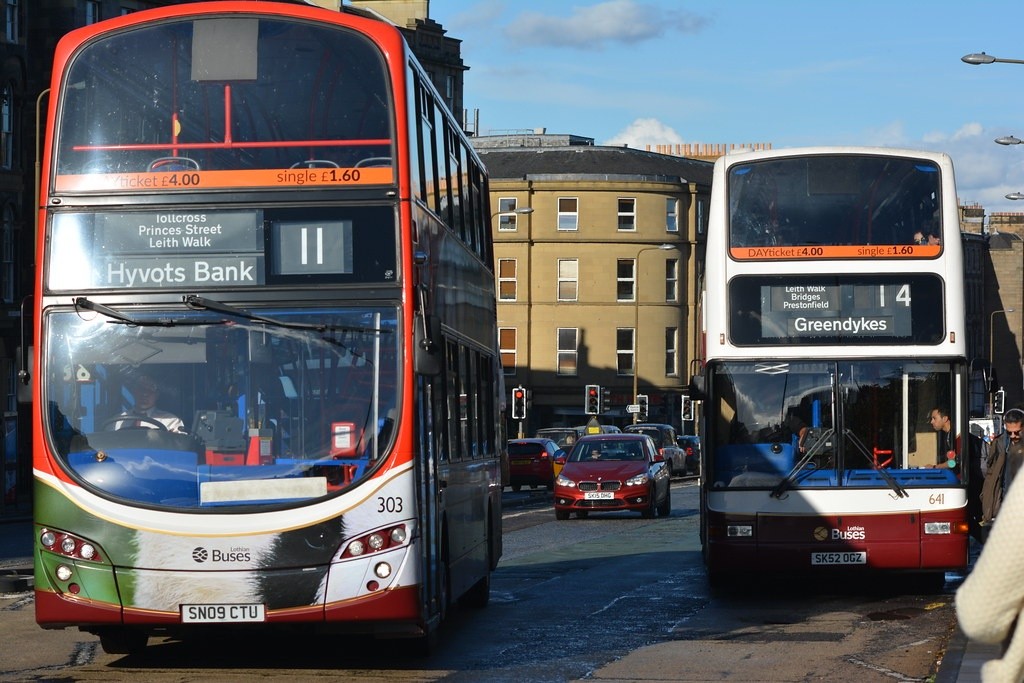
[
  {"x1": 16, "y1": 0, "x2": 508, "y2": 658},
  {"x1": 686, "y1": 145, "x2": 997, "y2": 588}
]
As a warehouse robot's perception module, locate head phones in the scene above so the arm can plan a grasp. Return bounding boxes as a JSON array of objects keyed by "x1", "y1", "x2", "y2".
[{"x1": 1004, "y1": 408, "x2": 1024, "y2": 430}]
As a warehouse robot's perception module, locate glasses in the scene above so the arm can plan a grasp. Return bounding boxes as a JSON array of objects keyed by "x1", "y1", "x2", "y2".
[{"x1": 1006, "y1": 429, "x2": 1021, "y2": 436}]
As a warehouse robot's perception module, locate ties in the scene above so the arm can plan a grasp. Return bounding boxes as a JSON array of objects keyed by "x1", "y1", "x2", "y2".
[{"x1": 132, "y1": 411, "x2": 146, "y2": 427}]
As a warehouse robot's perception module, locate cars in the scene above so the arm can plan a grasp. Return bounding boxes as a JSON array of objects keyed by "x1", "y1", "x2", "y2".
[
  {"x1": 507, "y1": 438, "x2": 566, "y2": 493},
  {"x1": 675, "y1": 434, "x2": 700, "y2": 477},
  {"x1": 536, "y1": 425, "x2": 623, "y2": 458},
  {"x1": 553, "y1": 434, "x2": 672, "y2": 520}
]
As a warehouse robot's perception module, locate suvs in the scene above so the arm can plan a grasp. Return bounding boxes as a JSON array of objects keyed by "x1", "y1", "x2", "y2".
[{"x1": 623, "y1": 422, "x2": 690, "y2": 478}]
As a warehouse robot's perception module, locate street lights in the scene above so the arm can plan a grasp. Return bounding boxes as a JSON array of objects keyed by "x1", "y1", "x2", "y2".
[{"x1": 989, "y1": 308, "x2": 1016, "y2": 418}]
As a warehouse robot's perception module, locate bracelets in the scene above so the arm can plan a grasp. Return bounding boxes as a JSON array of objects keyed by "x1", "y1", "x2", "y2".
[{"x1": 933, "y1": 465, "x2": 936, "y2": 469}]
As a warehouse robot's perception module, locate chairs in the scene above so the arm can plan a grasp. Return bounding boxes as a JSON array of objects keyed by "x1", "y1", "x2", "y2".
[
  {"x1": 129, "y1": 372, "x2": 181, "y2": 418},
  {"x1": 622, "y1": 444, "x2": 640, "y2": 461}
]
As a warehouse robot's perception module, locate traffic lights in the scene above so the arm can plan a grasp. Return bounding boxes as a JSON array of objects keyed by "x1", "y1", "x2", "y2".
[
  {"x1": 636, "y1": 395, "x2": 647, "y2": 422},
  {"x1": 994, "y1": 392, "x2": 1003, "y2": 412},
  {"x1": 585, "y1": 385, "x2": 599, "y2": 415},
  {"x1": 512, "y1": 388, "x2": 526, "y2": 419},
  {"x1": 681, "y1": 395, "x2": 694, "y2": 420}
]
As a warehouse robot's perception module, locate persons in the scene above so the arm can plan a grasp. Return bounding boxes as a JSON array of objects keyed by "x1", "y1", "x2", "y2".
[
  {"x1": 589, "y1": 449, "x2": 602, "y2": 461},
  {"x1": 979, "y1": 408, "x2": 1024, "y2": 527},
  {"x1": 564, "y1": 435, "x2": 575, "y2": 445},
  {"x1": 782, "y1": 413, "x2": 809, "y2": 453},
  {"x1": 103, "y1": 373, "x2": 187, "y2": 438},
  {"x1": 912, "y1": 217, "x2": 941, "y2": 245},
  {"x1": 925, "y1": 407, "x2": 990, "y2": 544}
]
